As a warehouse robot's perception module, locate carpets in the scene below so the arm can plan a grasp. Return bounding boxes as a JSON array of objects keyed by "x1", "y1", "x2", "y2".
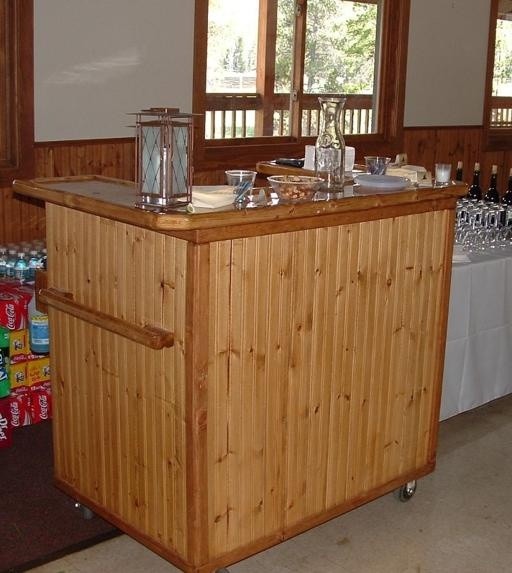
[{"x1": 0, "y1": 420, "x2": 124, "y2": 573}]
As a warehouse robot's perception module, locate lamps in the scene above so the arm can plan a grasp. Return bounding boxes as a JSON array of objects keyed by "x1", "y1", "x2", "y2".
[{"x1": 126, "y1": 108, "x2": 205, "y2": 214}]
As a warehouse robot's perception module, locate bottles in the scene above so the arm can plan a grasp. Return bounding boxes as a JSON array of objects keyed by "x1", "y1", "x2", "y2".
[
  {"x1": 314, "y1": 98, "x2": 345, "y2": 192},
  {"x1": 0, "y1": 238, "x2": 47, "y2": 289},
  {"x1": 455, "y1": 160, "x2": 512, "y2": 237}
]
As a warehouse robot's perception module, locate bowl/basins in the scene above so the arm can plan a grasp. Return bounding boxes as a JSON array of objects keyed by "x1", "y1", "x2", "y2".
[{"x1": 266, "y1": 173, "x2": 326, "y2": 200}]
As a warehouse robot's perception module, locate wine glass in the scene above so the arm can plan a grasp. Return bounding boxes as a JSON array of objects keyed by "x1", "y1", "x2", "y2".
[{"x1": 454, "y1": 198, "x2": 512, "y2": 255}]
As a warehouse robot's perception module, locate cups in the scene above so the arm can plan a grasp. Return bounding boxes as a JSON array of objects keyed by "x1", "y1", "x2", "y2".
[
  {"x1": 435, "y1": 163, "x2": 453, "y2": 186},
  {"x1": 364, "y1": 156, "x2": 393, "y2": 176},
  {"x1": 224, "y1": 168, "x2": 258, "y2": 209}
]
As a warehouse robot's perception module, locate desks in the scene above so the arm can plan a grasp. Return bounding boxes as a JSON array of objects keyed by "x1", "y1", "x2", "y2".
[{"x1": 438, "y1": 243, "x2": 512, "y2": 422}]
